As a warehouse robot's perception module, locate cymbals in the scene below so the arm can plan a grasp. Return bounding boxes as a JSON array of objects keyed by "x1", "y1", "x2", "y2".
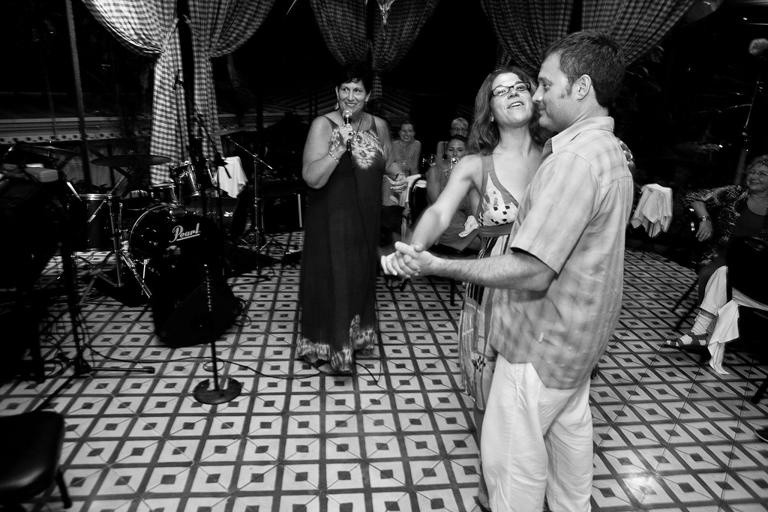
[
  {"x1": 92, "y1": 154, "x2": 173, "y2": 166},
  {"x1": 0, "y1": 140, "x2": 88, "y2": 163}
]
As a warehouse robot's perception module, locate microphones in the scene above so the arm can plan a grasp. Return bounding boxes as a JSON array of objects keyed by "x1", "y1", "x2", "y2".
[{"x1": 342, "y1": 108, "x2": 354, "y2": 152}]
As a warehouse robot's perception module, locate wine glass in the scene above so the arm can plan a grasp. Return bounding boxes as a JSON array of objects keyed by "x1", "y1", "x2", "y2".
[{"x1": 417, "y1": 153, "x2": 436, "y2": 171}]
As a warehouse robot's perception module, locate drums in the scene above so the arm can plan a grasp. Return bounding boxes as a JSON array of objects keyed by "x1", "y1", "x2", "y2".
[
  {"x1": 149, "y1": 183, "x2": 175, "y2": 205},
  {"x1": 79, "y1": 192, "x2": 112, "y2": 237},
  {"x1": 128, "y1": 206, "x2": 176, "y2": 260},
  {"x1": 169, "y1": 162, "x2": 196, "y2": 199}
]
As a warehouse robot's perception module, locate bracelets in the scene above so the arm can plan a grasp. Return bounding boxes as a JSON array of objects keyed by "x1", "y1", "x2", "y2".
[
  {"x1": 328, "y1": 150, "x2": 340, "y2": 165},
  {"x1": 697, "y1": 215, "x2": 711, "y2": 225}
]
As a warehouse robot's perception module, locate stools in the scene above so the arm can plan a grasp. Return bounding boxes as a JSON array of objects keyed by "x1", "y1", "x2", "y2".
[{"x1": 0, "y1": 407, "x2": 73, "y2": 511}]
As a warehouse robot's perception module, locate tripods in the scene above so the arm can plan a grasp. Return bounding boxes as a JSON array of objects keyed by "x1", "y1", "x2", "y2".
[
  {"x1": 226, "y1": 136, "x2": 297, "y2": 275},
  {"x1": 31, "y1": 196, "x2": 155, "y2": 413},
  {"x1": 42, "y1": 176, "x2": 153, "y2": 306}
]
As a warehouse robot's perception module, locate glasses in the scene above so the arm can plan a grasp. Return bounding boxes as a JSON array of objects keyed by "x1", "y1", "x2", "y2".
[{"x1": 490, "y1": 82, "x2": 531, "y2": 97}]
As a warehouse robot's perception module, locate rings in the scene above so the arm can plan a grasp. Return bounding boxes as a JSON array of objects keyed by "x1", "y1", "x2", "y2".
[{"x1": 627, "y1": 153, "x2": 634, "y2": 161}]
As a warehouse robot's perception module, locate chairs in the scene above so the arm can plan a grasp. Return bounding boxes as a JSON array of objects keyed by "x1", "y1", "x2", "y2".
[
  {"x1": 672, "y1": 210, "x2": 723, "y2": 330},
  {"x1": 398, "y1": 176, "x2": 459, "y2": 307}
]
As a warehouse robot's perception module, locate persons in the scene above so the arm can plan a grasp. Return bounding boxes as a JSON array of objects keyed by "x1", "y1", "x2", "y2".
[
  {"x1": 295, "y1": 59, "x2": 410, "y2": 379},
  {"x1": 373, "y1": 120, "x2": 422, "y2": 251},
  {"x1": 436, "y1": 116, "x2": 471, "y2": 162},
  {"x1": 424, "y1": 134, "x2": 481, "y2": 255},
  {"x1": 665, "y1": 153, "x2": 767, "y2": 357}
]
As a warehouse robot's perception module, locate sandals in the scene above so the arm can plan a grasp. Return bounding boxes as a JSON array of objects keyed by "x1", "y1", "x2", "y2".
[{"x1": 663, "y1": 330, "x2": 709, "y2": 353}]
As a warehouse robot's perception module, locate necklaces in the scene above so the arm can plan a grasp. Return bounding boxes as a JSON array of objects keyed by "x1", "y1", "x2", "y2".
[{"x1": 355, "y1": 118, "x2": 362, "y2": 133}]
